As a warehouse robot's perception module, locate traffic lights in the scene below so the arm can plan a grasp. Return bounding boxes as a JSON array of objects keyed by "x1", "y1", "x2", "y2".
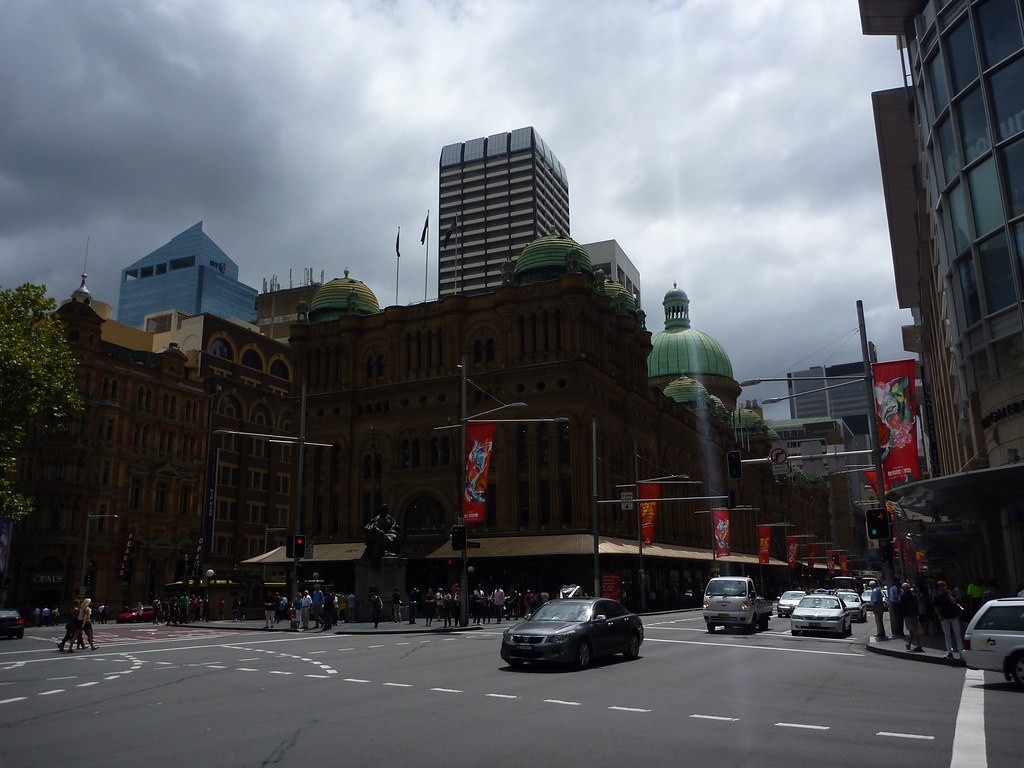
[
  {"x1": 727, "y1": 450, "x2": 743, "y2": 480},
  {"x1": 293, "y1": 535, "x2": 306, "y2": 558},
  {"x1": 866, "y1": 509, "x2": 889, "y2": 539}
]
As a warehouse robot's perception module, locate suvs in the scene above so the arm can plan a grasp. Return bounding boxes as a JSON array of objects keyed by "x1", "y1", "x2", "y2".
[{"x1": 960, "y1": 596, "x2": 1024, "y2": 689}]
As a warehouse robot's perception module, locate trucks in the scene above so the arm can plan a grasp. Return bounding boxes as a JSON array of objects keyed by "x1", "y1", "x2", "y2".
[{"x1": 702, "y1": 574, "x2": 773, "y2": 633}]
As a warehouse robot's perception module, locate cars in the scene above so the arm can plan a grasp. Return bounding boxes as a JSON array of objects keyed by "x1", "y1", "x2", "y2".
[
  {"x1": 815, "y1": 576, "x2": 889, "y2": 623},
  {"x1": 0, "y1": 608, "x2": 24, "y2": 639},
  {"x1": 790, "y1": 594, "x2": 852, "y2": 636},
  {"x1": 499, "y1": 597, "x2": 645, "y2": 671},
  {"x1": 777, "y1": 590, "x2": 807, "y2": 618},
  {"x1": 116, "y1": 605, "x2": 164, "y2": 622}
]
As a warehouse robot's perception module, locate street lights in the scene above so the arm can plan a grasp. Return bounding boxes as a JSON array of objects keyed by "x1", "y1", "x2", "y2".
[
  {"x1": 756, "y1": 522, "x2": 796, "y2": 574},
  {"x1": 739, "y1": 376, "x2": 897, "y2": 587},
  {"x1": 460, "y1": 401, "x2": 571, "y2": 626},
  {"x1": 79, "y1": 513, "x2": 119, "y2": 602},
  {"x1": 635, "y1": 473, "x2": 705, "y2": 613},
  {"x1": 694, "y1": 503, "x2": 761, "y2": 578},
  {"x1": 212, "y1": 429, "x2": 305, "y2": 628},
  {"x1": 785, "y1": 531, "x2": 860, "y2": 577},
  {"x1": 207, "y1": 569, "x2": 214, "y2": 622}
]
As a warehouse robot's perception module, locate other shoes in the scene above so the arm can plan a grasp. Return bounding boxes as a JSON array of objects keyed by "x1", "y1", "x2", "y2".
[
  {"x1": 67, "y1": 650, "x2": 75, "y2": 653},
  {"x1": 57, "y1": 643, "x2": 65, "y2": 651},
  {"x1": 91, "y1": 647, "x2": 99, "y2": 651},
  {"x1": 82, "y1": 646, "x2": 89, "y2": 650},
  {"x1": 313, "y1": 626, "x2": 319, "y2": 629}
]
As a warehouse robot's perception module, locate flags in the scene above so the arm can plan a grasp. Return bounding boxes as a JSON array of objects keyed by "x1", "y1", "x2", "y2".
[
  {"x1": 421, "y1": 215, "x2": 429, "y2": 244},
  {"x1": 396, "y1": 233, "x2": 401, "y2": 258},
  {"x1": 441, "y1": 218, "x2": 457, "y2": 249}
]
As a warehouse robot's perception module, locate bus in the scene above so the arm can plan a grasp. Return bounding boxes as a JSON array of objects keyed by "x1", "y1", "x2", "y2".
[{"x1": 164, "y1": 579, "x2": 287, "y2": 621}]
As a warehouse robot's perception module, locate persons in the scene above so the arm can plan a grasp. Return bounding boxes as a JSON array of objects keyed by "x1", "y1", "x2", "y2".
[
  {"x1": 133, "y1": 583, "x2": 561, "y2": 631},
  {"x1": 868, "y1": 576, "x2": 984, "y2": 658},
  {"x1": 786, "y1": 579, "x2": 824, "y2": 594},
  {"x1": 32, "y1": 598, "x2": 109, "y2": 653}
]
